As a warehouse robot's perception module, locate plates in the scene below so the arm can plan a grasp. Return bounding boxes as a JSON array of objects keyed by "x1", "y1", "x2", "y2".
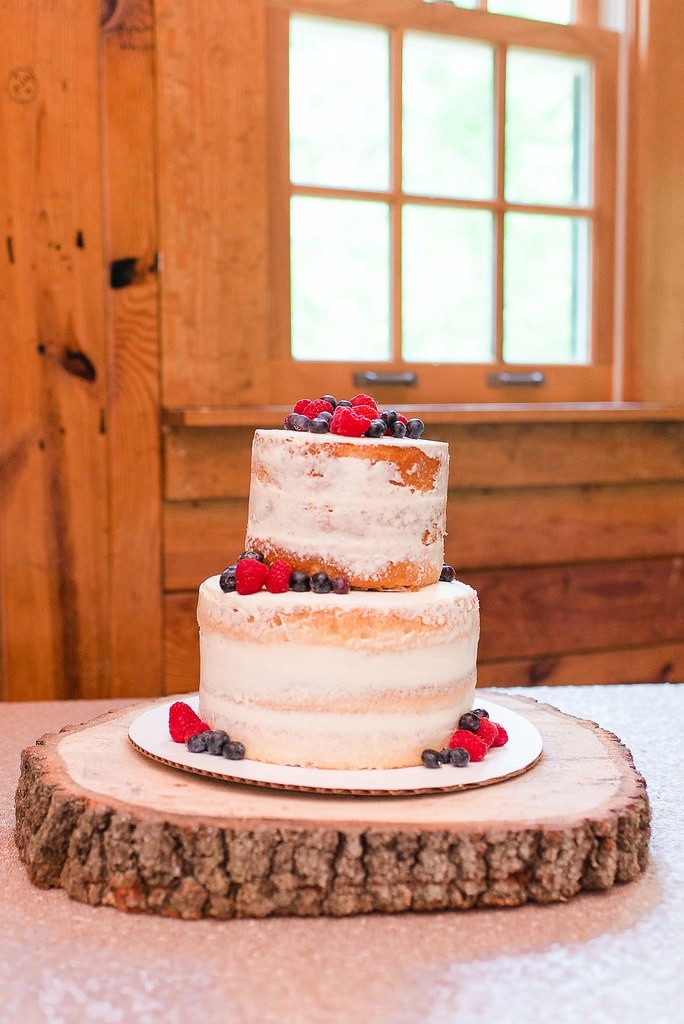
[{"x1": 126, "y1": 694, "x2": 543, "y2": 796}]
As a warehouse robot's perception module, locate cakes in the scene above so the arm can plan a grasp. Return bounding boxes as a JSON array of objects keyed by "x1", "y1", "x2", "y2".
[{"x1": 170, "y1": 393, "x2": 510, "y2": 768}]
